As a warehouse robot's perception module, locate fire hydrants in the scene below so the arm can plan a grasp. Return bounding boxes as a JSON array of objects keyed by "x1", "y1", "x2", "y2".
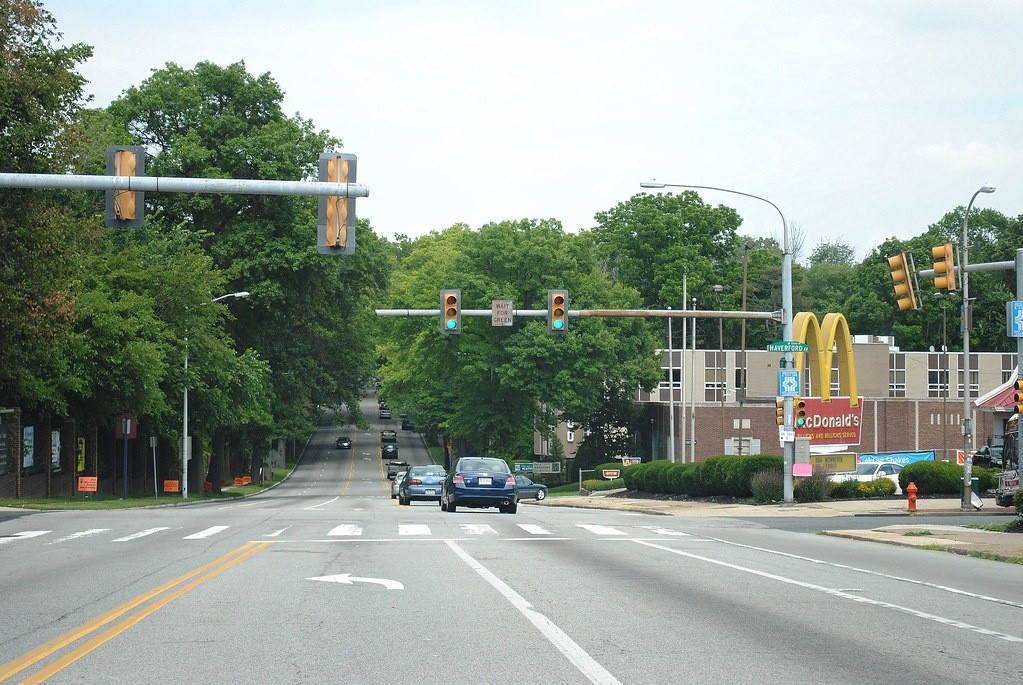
[{"x1": 906, "y1": 482, "x2": 918, "y2": 511}]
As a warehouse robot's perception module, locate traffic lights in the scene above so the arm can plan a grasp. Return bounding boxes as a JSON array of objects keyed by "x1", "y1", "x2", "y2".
[
  {"x1": 886, "y1": 252, "x2": 917, "y2": 311},
  {"x1": 548, "y1": 290, "x2": 569, "y2": 334},
  {"x1": 932, "y1": 244, "x2": 956, "y2": 291},
  {"x1": 775, "y1": 399, "x2": 784, "y2": 425},
  {"x1": 795, "y1": 399, "x2": 806, "y2": 428},
  {"x1": 1014, "y1": 380, "x2": 1023, "y2": 414},
  {"x1": 440, "y1": 289, "x2": 461, "y2": 334}
]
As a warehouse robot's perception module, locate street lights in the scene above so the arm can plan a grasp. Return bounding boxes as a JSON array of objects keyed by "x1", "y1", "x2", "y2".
[
  {"x1": 738, "y1": 240, "x2": 756, "y2": 458},
  {"x1": 933, "y1": 292, "x2": 956, "y2": 460},
  {"x1": 640, "y1": 177, "x2": 794, "y2": 503},
  {"x1": 712, "y1": 284, "x2": 732, "y2": 455},
  {"x1": 960, "y1": 182, "x2": 997, "y2": 511},
  {"x1": 181, "y1": 291, "x2": 251, "y2": 500}
]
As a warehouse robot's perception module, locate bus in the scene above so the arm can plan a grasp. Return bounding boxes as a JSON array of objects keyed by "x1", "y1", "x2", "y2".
[{"x1": 987, "y1": 413, "x2": 1020, "y2": 507}]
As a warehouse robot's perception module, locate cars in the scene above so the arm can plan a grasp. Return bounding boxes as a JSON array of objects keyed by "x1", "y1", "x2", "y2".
[
  {"x1": 380, "y1": 409, "x2": 391, "y2": 419},
  {"x1": 336, "y1": 436, "x2": 352, "y2": 450},
  {"x1": 441, "y1": 457, "x2": 518, "y2": 515},
  {"x1": 385, "y1": 458, "x2": 410, "y2": 479},
  {"x1": 972, "y1": 445, "x2": 1009, "y2": 469},
  {"x1": 381, "y1": 443, "x2": 399, "y2": 459},
  {"x1": 401, "y1": 419, "x2": 415, "y2": 430},
  {"x1": 389, "y1": 470, "x2": 407, "y2": 499},
  {"x1": 828, "y1": 462, "x2": 905, "y2": 495},
  {"x1": 378, "y1": 396, "x2": 390, "y2": 410},
  {"x1": 514, "y1": 475, "x2": 549, "y2": 503},
  {"x1": 381, "y1": 429, "x2": 398, "y2": 443},
  {"x1": 398, "y1": 464, "x2": 448, "y2": 505}
]
